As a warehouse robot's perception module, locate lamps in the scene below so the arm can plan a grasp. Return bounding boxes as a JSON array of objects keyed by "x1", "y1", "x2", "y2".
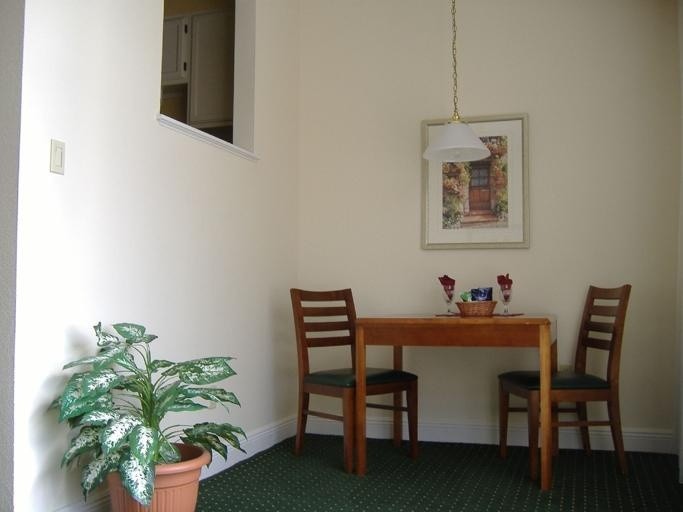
[{"x1": 421, "y1": 0, "x2": 490, "y2": 164}]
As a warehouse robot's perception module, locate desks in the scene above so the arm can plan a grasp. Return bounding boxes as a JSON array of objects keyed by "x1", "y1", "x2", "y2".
[{"x1": 355, "y1": 314, "x2": 550, "y2": 491}]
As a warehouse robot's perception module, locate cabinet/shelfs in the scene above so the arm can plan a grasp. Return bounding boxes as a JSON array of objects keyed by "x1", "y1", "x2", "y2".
[
  {"x1": 161, "y1": 18, "x2": 188, "y2": 86},
  {"x1": 188, "y1": 8, "x2": 232, "y2": 125}
]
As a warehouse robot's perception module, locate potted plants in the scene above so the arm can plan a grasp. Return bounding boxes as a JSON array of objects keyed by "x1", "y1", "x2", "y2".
[{"x1": 48, "y1": 319, "x2": 247, "y2": 512}]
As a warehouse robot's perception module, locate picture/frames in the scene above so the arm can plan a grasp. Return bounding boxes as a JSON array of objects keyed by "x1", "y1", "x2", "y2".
[{"x1": 420, "y1": 113, "x2": 529, "y2": 250}]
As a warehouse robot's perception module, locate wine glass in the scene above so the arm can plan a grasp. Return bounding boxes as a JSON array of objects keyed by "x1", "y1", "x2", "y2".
[
  {"x1": 496, "y1": 285, "x2": 513, "y2": 316},
  {"x1": 440, "y1": 285, "x2": 456, "y2": 314}
]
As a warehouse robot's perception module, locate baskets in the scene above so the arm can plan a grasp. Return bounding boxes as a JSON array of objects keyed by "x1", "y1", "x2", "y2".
[{"x1": 454, "y1": 301, "x2": 497, "y2": 317}]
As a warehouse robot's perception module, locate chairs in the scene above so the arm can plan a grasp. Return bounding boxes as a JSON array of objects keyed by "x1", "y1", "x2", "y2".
[
  {"x1": 498, "y1": 285, "x2": 631, "y2": 480},
  {"x1": 290, "y1": 288, "x2": 418, "y2": 474}
]
